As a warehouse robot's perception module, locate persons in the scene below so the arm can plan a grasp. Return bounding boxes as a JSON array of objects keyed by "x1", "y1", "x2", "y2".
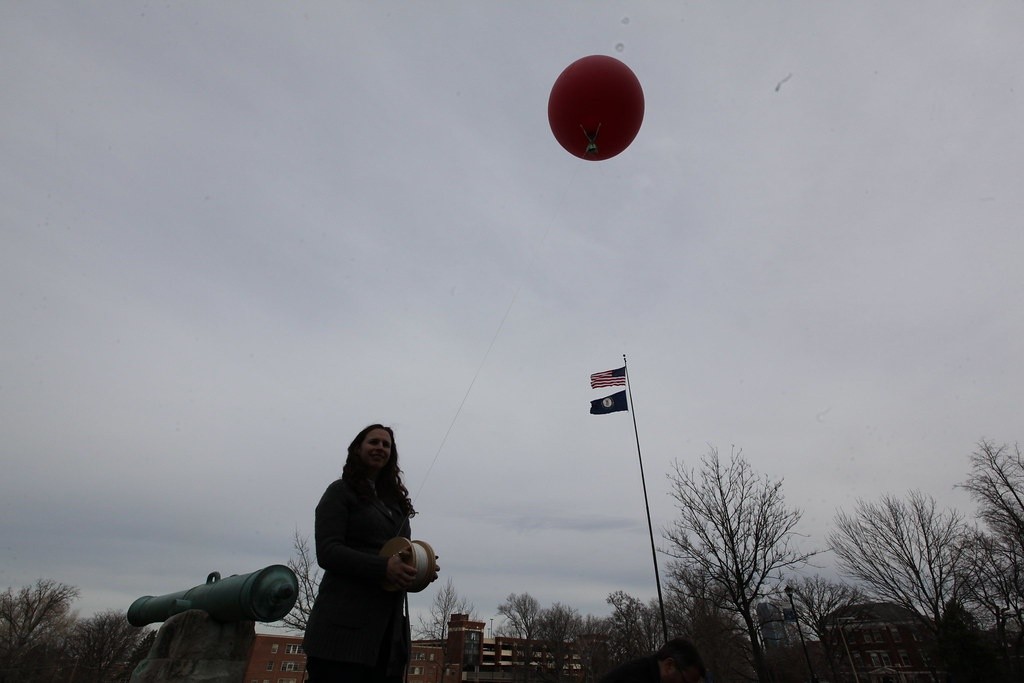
[
  {"x1": 596, "y1": 637, "x2": 707, "y2": 683},
  {"x1": 300, "y1": 423, "x2": 440, "y2": 683}
]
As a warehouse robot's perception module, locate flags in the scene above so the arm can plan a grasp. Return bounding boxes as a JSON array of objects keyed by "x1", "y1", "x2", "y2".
[
  {"x1": 590, "y1": 367, "x2": 627, "y2": 390},
  {"x1": 589, "y1": 390, "x2": 628, "y2": 414}
]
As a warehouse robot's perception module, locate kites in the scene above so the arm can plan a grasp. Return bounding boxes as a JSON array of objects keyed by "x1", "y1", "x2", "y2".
[{"x1": 548, "y1": 55, "x2": 646, "y2": 163}]
